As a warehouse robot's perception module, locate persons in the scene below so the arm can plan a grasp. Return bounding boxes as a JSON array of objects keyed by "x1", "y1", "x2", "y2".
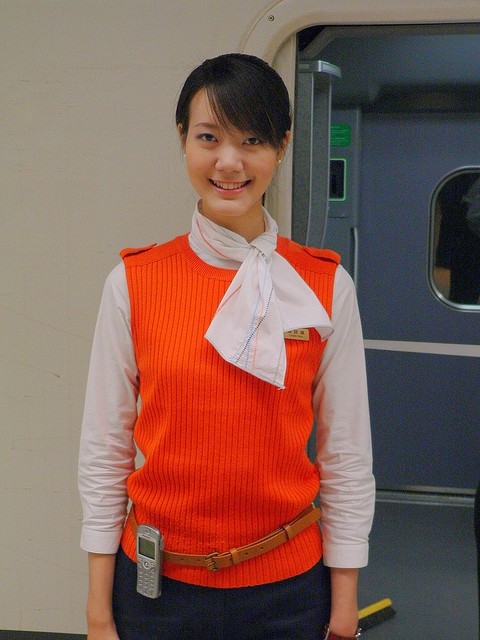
[{"x1": 78, "y1": 54, "x2": 376, "y2": 640}]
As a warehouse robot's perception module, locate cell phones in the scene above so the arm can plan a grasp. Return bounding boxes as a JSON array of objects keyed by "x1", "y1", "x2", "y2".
[{"x1": 134, "y1": 523, "x2": 164, "y2": 600}]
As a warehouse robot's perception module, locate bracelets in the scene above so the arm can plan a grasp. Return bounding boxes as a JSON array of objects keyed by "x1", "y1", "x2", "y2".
[{"x1": 324, "y1": 624, "x2": 361, "y2": 640}]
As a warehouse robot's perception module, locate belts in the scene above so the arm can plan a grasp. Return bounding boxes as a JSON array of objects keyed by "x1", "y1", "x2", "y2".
[{"x1": 127, "y1": 505, "x2": 322, "y2": 572}]
